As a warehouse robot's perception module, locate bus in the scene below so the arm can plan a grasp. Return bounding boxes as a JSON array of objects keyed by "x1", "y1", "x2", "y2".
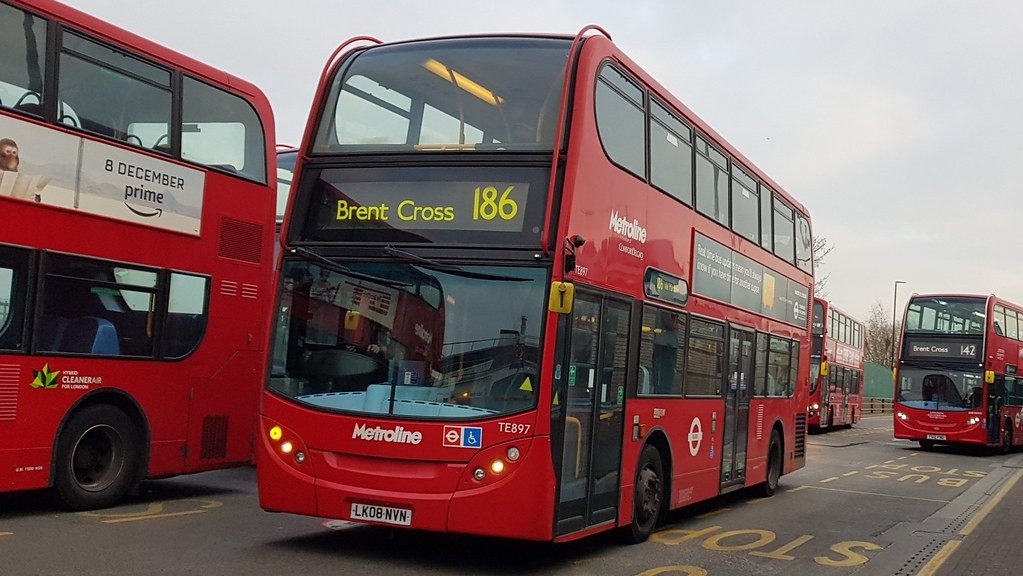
[
  {"x1": 0, "y1": 0, "x2": 276, "y2": 514},
  {"x1": 809, "y1": 298, "x2": 865, "y2": 430},
  {"x1": 254, "y1": 25, "x2": 813, "y2": 550},
  {"x1": 892, "y1": 293, "x2": 1023, "y2": 450}
]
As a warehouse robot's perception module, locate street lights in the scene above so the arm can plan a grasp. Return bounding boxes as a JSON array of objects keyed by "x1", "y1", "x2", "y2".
[{"x1": 892, "y1": 281, "x2": 908, "y2": 369}]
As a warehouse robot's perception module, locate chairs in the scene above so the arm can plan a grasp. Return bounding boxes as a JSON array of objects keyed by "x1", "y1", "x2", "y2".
[
  {"x1": 39, "y1": 315, "x2": 168, "y2": 356},
  {"x1": 488, "y1": 360, "x2": 652, "y2": 400},
  {"x1": 15, "y1": 91, "x2": 169, "y2": 154}
]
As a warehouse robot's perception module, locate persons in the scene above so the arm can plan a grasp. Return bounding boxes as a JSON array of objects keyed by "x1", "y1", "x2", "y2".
[
  {"x1": 345, "y1": 317, "x2": 408, "y2": 363},
  {"x1": 310, "y1": 268, "x2": 334, "y2": 302}
]
